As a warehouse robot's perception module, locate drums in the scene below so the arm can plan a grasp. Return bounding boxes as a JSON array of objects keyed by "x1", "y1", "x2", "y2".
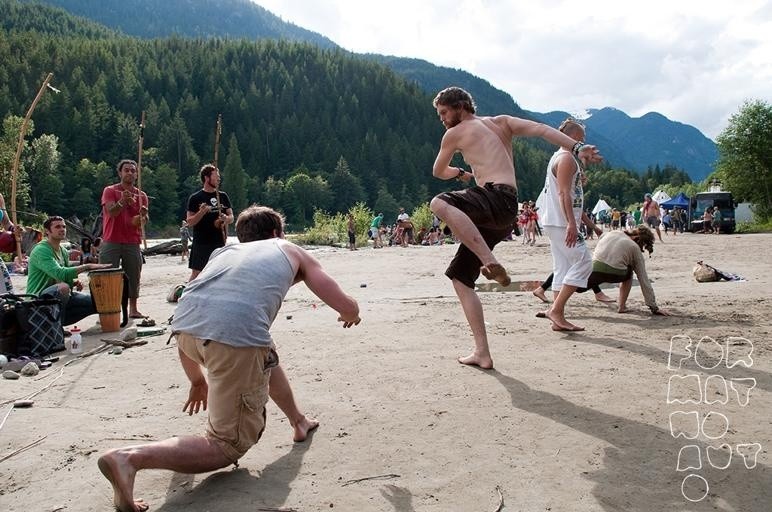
[{"x1": 89, "y1": 267, "x2": 124, "y2": 332}]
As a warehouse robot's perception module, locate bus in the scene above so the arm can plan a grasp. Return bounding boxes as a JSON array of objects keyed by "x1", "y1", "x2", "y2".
[{"x1": 690, "y1": 191, "x2": 738, "y2": 233}]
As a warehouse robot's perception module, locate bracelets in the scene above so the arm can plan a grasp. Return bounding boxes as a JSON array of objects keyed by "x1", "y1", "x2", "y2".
[
  {"x1": 454, "y1": 167, "x2": 465, "y2": 180},
  {"x1": 117, "y1": 201, "x2": 124, "y2": 208},
  {"x1": 571, "y1": 140, "x2": 584, "y2": 157}
]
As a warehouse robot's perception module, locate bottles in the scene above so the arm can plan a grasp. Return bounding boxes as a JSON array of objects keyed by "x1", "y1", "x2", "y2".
[{"x1": 70, "y1": 325, "x2": 82, "y2": 354}]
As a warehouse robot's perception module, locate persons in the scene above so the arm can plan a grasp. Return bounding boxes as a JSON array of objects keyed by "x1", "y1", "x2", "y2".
[
  {"x1": 576, "y1": 225, "x2": 672, "y2": 316},
  {"x1": 97, "y1": 160, "x2": 150, "y2": 320},
  {"x1": 96, "y1": 205, "x2": 362, "y2": 512},
  {"x1": 27, "y1": 216, "x2": 112, "y2": 337},
  {"x1": 534, "y1": 117, "x2": 593, "y2": 332},
  {"x1": 185, "y1": 164, "x2": 234, "y2": 282},
  {"x1": 430, "y1": 86, "x2": 604, "y2": 368},
  {"x1": 179, "y1": 220, "x2": 192, "y2": 262},
  {"x1": 523, "y1": 168, "x2": 618, "y2": 304},
  {"x1": 0, "y1": 193, "x2": 25, "y2": 290},
  {"x1": 345, "y1": 192, "x2": 721, "y2": 252},
  {"x1": 11, "y1": 216, "x2": 103, "y2": 274}
]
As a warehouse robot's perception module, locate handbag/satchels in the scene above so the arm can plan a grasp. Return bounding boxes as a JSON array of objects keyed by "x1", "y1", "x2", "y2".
[{"x1": 0, "y1": 292, "x2": 66, "y2": 358}]
